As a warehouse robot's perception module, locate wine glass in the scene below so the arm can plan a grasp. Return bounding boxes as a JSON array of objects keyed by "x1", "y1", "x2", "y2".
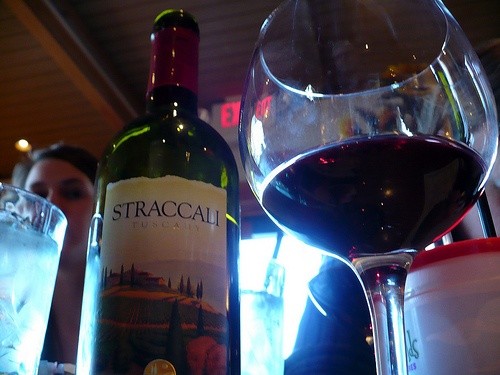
[{"x1": 235, "y1": 2, "x2": 499, "y2": 375}]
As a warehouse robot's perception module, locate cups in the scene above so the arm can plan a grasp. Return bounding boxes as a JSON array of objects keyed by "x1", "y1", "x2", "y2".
[
  {"x1": 235, "y1": 256, "x2": 283, "y2": 375},
  {"x1": 0, "y1": 181, "x2": 68, "y2": 375}
]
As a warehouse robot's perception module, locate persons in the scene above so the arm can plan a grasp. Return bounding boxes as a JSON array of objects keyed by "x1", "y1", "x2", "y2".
[
  {"x1": 11, "y1": 140, "x2": 102, "y2": 374},
  {"x1": 284, "y1": 248, "x2": 378, "y2": 375}
]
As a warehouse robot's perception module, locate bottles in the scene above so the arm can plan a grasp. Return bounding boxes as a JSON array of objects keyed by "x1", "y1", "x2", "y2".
[
  {"x1": 405, "y1": 234, "x2": 500, "y2": 375},
  {"x1": 74, "y1": 7, "x2": 238, "y2": 375}
]
